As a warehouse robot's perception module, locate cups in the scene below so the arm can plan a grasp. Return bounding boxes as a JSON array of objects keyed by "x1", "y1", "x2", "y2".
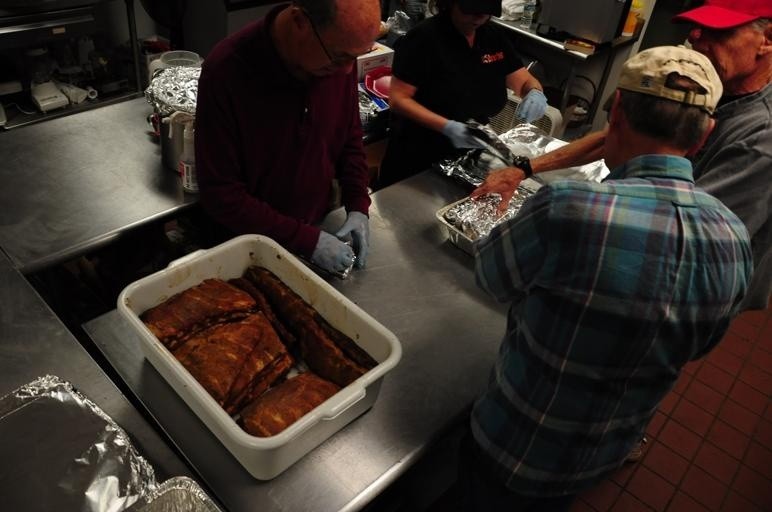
[{"x1": 148, "y1": 50, "x2": 205, "y2": 87}]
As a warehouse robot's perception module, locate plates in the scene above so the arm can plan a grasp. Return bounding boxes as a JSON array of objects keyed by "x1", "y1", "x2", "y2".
[{"x1": 364, "y1": 66, "x2": 392, "y2": 100}]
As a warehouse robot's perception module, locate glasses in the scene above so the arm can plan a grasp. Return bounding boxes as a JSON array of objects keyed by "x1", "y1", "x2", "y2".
[{"x1": 298, "y1": 6, "x2": 378, "y2": 69}]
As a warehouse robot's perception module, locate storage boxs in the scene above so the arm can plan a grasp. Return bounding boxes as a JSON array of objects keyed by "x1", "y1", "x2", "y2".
[
  {"x1": 116, "y1": 233, "x2": 402, "y2": 481},
  {"x1": 436, "y1": 182, "x2": 535, "y2": 256},
  {"x1": 356, "y1": 41, "x2": 395, "y2": 81}
]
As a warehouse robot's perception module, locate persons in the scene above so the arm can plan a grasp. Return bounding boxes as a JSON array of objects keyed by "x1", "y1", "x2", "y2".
[
  {"x1": 194, "y1": 1, "x2": 382, "y2": 277},
  {"x1": 470, "y1": 44, "x2": 755, "y2": 512},
  {"x1": 376, "y1": 0, "x2": 549, "y2": 188},
  {"x1": 471, "y1": 0, "x2": 772, "y2": 313}
]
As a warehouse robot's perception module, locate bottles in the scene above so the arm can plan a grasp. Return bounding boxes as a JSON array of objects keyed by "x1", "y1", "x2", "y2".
[
  {"x1": 178, "y1": 127, "x2": 202, "y2": 195},
  {"x1": 520, "y1": 0, "x2": 536, "y2": 29}
]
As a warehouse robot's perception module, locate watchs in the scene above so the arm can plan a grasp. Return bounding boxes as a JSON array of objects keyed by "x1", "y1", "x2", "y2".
[{"x1": 514, "y1": 155, "x2": 532, "y2": 179}]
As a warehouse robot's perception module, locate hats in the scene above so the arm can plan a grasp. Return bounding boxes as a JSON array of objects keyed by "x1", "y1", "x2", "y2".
[
  {"x1": 672, "y1": 0, "x2": 772, "y2": 31},
  {"x1": 601, "y1": 45, "x2": 724, "y2": 117}
]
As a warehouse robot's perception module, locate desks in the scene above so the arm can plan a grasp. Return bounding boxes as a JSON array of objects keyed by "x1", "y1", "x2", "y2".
[{"x1": 491, "y1": 16, "x2": 639, "y2": 127}]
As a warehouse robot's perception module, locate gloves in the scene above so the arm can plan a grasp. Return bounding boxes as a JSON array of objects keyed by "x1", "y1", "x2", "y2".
[
  {"x1": 516, "y1": 88, "x2": 548, "y2": 124},
  {"x1": 443, "y1": 120, "x2": 496, "y2": 149},
  {"x1": 312, "y1": 210, "x2": 370, "y2": 274}
]
